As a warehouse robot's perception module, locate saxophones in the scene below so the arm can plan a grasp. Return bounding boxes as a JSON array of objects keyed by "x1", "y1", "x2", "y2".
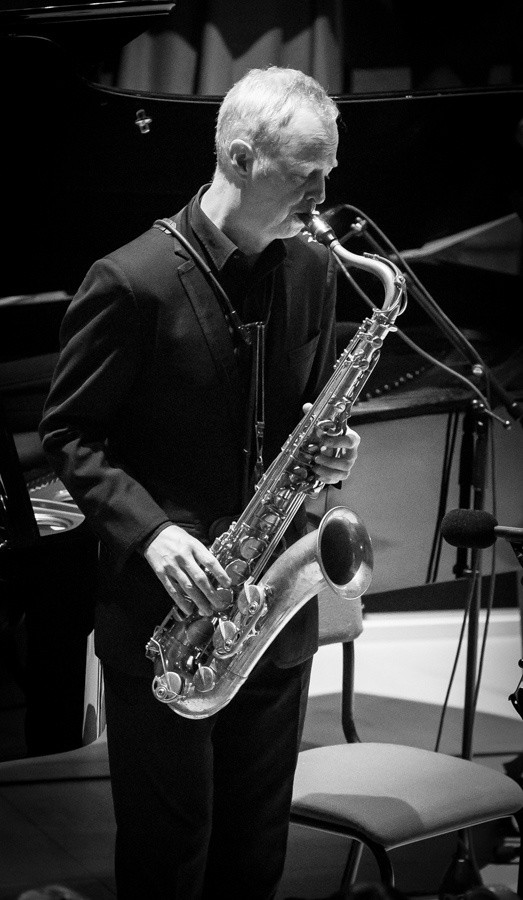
[{"x1": 144, "y1": 215, "x2": 412, "y2": 722}]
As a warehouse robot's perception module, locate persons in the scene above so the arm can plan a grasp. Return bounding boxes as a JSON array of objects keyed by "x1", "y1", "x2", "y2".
[{"x1": 40, "y1": 69, "x2": 361, "y2": 900}]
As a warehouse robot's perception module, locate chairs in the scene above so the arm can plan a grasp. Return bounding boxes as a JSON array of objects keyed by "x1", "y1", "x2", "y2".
[{"x1": 288, "y1": 587, "x2": 521, "y2": 900}]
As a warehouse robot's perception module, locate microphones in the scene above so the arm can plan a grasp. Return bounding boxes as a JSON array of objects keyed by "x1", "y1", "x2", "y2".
[{"x1": 438, "y1": 507, "x2": 523, "y2": 554}]
